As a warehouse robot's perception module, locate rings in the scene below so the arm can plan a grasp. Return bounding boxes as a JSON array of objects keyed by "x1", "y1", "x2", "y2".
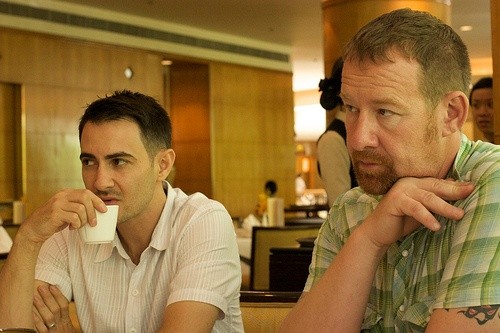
[{"x1": 49, "y1": 322, "x2": 56, "y2": 328}]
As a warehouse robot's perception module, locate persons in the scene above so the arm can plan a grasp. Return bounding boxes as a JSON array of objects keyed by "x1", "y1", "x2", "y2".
[
  {"x1": 469, "y1": 74, "x2": 495, "y2": 143},
  {"x1": 0, "y1": 88, "x2": 245, "y2": 333},
  {"x1": 315, "y1": 55, "x2": 360, "y2": 211},
  {"x1": 262, "y1": 181, "x2": 277, "y2": 226},
  {"x1": 295, "y1": 173, "x2": 306, "y2": 196},
  {"x1": 278, "y1": 8, "x2": 500, "y2": 333}
]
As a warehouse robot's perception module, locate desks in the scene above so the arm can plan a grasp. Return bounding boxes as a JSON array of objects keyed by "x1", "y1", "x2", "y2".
[
  {"x1": 294, "y1": 236, "x2": 316, "y2": 248},
  {"x1": 284, "y1": 217, "x2": 326, "y2": 227}
]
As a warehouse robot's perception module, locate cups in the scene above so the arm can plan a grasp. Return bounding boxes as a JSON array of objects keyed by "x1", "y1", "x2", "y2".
[
  {"x1": 78, "y1": 205, "x2": 119, "y2": 244},
  {"x1": 268, "y1": 198, "x2": 284, "y2": 227}
]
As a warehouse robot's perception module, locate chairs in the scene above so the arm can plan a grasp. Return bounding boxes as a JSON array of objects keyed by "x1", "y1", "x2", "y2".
[{"x1": 268, "y1": 246, "x2": 314, "y2": 293}]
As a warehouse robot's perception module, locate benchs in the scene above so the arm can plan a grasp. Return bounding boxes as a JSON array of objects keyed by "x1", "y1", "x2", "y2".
[{"x1": 69, "y1": 291, "x2": 302, "y2": 333}]
